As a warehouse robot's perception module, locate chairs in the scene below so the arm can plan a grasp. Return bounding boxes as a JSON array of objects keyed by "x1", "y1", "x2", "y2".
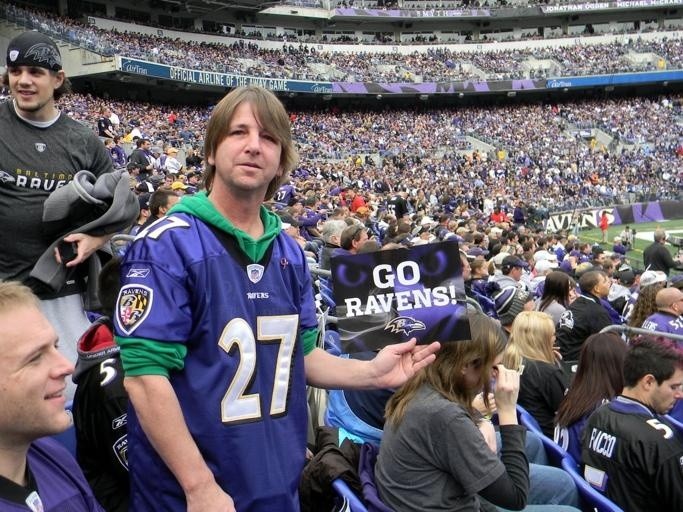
[
  {"x1": 519, "y1": 413, "x2": 578, "y2": 468},
  {"x1": 490, "y1": 382, "x2": 542, "y2": 431},
  {"x1": 663, "y1": 413, "x2": 683, "y2": 430},
  {"x1": 48, "y1": 400, "x2": 77, "y2": 457},
  {"x1": 560, "y1": 457, "x2": 625, "y2": 512},
  {"x1": 332, "y1": 478, "x2": 367, "y2": 512}
]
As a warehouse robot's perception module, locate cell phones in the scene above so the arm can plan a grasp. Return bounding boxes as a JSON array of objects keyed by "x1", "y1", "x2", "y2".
[{"x1": 56, "y1": 238, "x2": 77, "y2": 264}]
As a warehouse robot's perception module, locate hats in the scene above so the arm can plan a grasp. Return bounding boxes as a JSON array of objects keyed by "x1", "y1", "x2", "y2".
[
  {"x1": 456, "y1": 227, "x2": 470, "y2": 233},
  {"x1": 503, "y1": 256, "x2": 530, "y2": 267},
  {"x1": 614, "y1": 236, "x2": 622, "y2": 242},
  {"x1": 467, "y1": 247, "x2": 490, "y2": 256},
  {"x1": 494, "y1": 286, "x2": 529, "y2": 324},
  {"x1": 535, "y1": 260, "x2": 558, "y2": 271},
  {"x1": 172, "y1": 181, "x2": 188, "y2": 189},
  {"x1": 492, "y1": 227, "x2": 503, "y2": 233},
  {"x1": 167, "y1": 148, "x2": 178, "y2": 153},
  {"x1": 640, "y1": 270, "x2": 667, "y2": 286},
  {"x1": 127, "y1": 162, "x2": 141, "y2": 169},
  {"x1": 494, "y1": 253, "x2": 510, "y2": 264},
  {"x1": 533, "y1": 250, "x2": 557, "y2": 260},
  {"x1": 6, "y1": 32, "x2": 62, "y2": 71}
]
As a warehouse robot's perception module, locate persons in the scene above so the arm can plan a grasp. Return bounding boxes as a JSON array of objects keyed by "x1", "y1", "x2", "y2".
[{"x1": 1, "y1": 0, "x2": 683, "y2": 511}]
